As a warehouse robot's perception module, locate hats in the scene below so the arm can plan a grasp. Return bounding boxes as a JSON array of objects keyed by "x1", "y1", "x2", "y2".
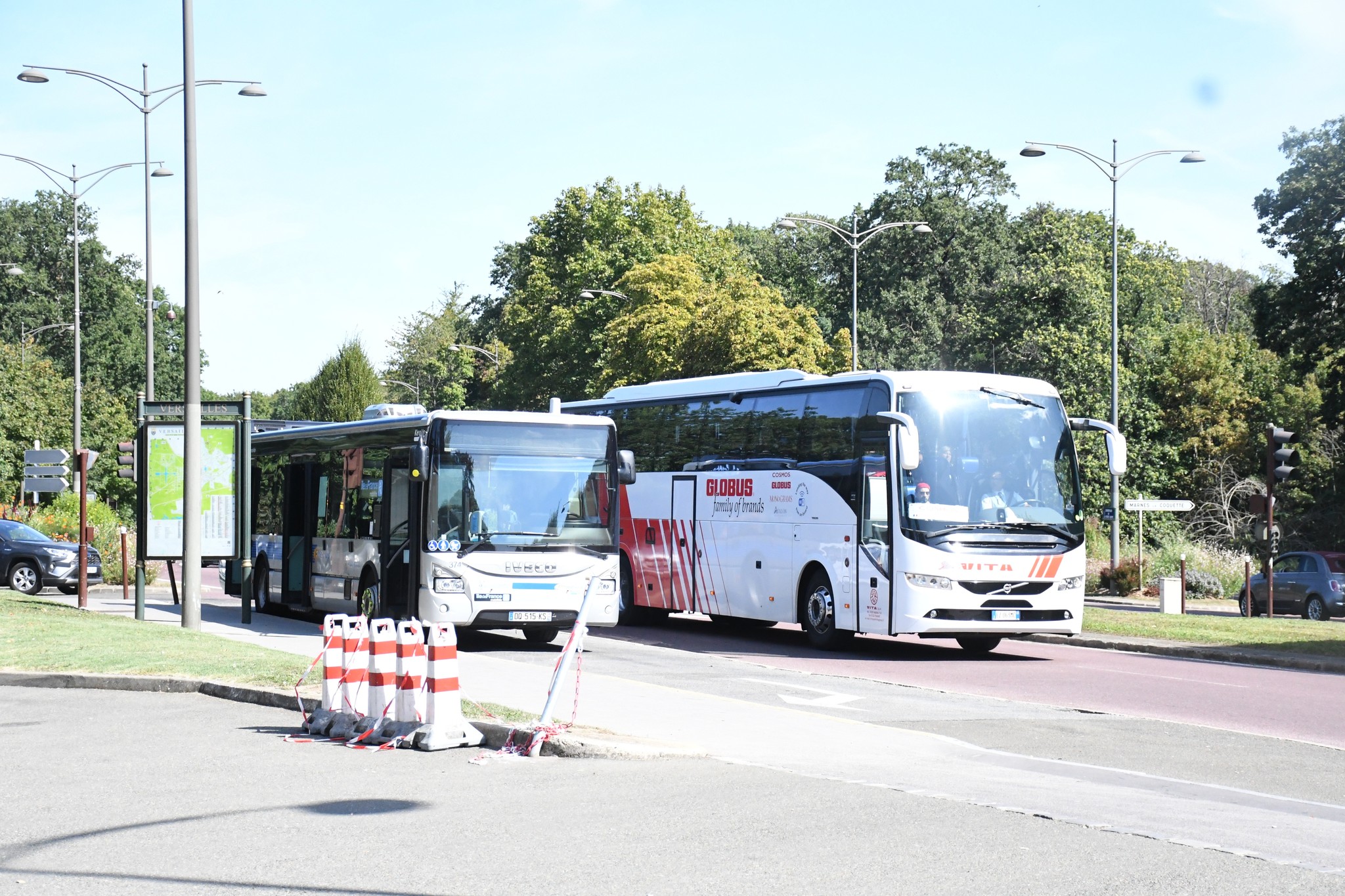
[{"x1": 916, "y1": 483, "x2": 930, "y2": 491}]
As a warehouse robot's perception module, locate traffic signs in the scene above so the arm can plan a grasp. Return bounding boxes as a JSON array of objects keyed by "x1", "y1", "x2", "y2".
[{"x1": 1124, "y1": 500, "x2": 1196, "y2": 510}]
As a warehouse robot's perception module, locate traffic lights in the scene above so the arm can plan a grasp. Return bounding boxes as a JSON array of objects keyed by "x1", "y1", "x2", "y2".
[
  {"x1": 1270, "y1": 427, "x2": 1301, "y2": 483},
  {"x1": 116, "y1": 438, "x2": 137, "y2": 483},
  {"x1": 82, "y1": 448, "x2": 99, "y2": 471},
  {"x1": 1270, "y1": 529, "x2": 1280, "y2": 553}
]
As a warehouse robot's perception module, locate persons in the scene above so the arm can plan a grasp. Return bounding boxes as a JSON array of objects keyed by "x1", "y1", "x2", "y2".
[{"x1": 902, "y1": 444, "x2": 1034, "y2": 524}]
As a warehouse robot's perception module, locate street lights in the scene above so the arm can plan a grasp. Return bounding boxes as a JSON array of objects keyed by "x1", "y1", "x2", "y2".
[
  {"x1": 776, "y1": 214, "x2": 933, "y2": 371},
  {"x1": 1, "y1": 153, "x2": 175, "y2": 492},
  {"x1": 448, "y1": 343, "x2": 499, "y2": 388},
  {"x1": 21, "y1": 321, "x2": 82, "y2": 371},
  {"x1": 1019, "y1": 137, "x2": 1206, "y2": 596},
  {"x1": 14, "y1": 62, "x2": 269, "y2": 418},
  {"x1": 379, "y1": 378, "x2": 419, "y2": 405}
]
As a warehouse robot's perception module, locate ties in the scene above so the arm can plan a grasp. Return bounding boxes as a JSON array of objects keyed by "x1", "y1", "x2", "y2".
[{"x1": 998, "y1": 492, "x2": 1007, "y2": 505}]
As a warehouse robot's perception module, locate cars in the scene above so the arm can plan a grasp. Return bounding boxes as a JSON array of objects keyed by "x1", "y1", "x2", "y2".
[
  {"x1": 0, "y1": 518, "x2": 104, "y2": 595},
  {"x1": 1239, "y1": 551, "x2": 1345, "y2": 620}
]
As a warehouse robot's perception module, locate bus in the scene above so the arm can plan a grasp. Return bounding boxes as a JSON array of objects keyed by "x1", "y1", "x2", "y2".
[
  {"x1": 221, "y1": 404, "x2": 636, "y2": 645},
  {"x1": 560, "y1": 367, "x2": 1127, "y2": 655}
]
